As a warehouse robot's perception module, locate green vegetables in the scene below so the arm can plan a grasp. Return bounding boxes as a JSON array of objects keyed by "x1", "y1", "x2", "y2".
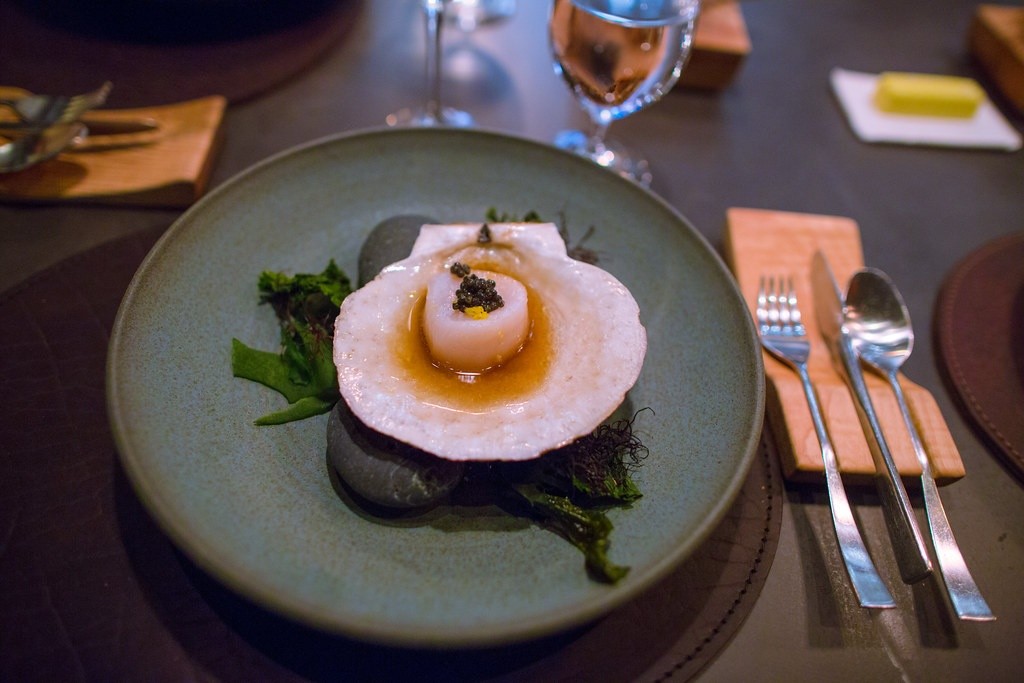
[{"x1": 231, "y1": 255, "x2": 644, "y2": 584}]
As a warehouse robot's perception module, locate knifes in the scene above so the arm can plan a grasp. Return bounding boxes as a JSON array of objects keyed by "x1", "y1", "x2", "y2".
[
  {"x1": 812, "y1": 252, "x2": 933, "y2": 586},
  {"x1": 0, "y1": 116, "x2": 158, "y2": 135}
]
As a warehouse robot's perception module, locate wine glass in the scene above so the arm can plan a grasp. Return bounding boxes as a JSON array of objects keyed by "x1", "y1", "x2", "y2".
[
  {"x1": 546, "y1": 0, "x2": 703, "y2": 187},
  {"x1": 386, "y1": 0, "x2": 475, "y2": 129}
]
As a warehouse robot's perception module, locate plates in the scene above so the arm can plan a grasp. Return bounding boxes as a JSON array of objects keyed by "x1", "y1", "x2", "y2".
[{"x1": 105, "y1": 125, "x2": 767, "y2": 644}]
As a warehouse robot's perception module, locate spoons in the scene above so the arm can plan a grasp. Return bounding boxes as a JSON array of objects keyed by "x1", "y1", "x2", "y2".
[
  {"x1": 845, "y1": 267, "x2": 996, "y2": 622},
  {"x1": 0, "y1": 121, "x2": 88, "y2": 174}
]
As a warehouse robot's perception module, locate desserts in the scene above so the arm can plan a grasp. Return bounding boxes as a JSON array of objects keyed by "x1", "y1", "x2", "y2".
[{"x1": 876, "y1": 71, "x2": 985, "y2": 119}]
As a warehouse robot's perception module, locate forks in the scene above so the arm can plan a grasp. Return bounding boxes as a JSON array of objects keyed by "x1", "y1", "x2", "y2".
[
  {"x1": 0, "y1": 80, "x2": 111, "y2": 124},
  {"x1": 757, "y1": 275, "x2": 897, "y2": 609}
]
